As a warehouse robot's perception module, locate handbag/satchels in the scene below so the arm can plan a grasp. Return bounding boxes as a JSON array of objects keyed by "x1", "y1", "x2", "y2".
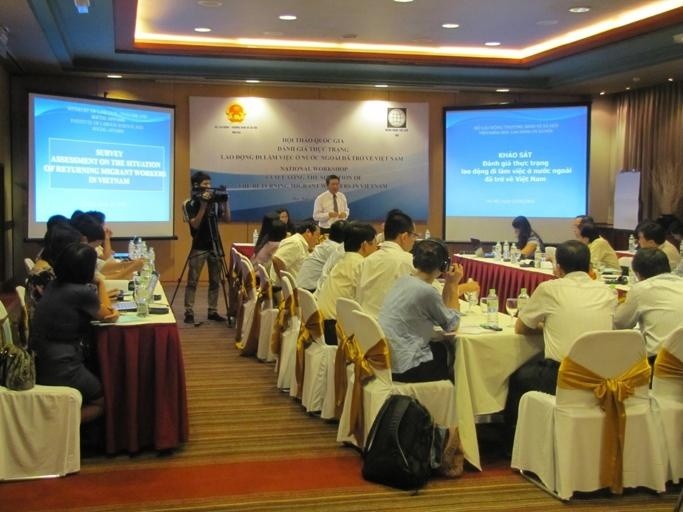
[{"x1": 0, "y1": 344, "x2": 37, "y2": 391}]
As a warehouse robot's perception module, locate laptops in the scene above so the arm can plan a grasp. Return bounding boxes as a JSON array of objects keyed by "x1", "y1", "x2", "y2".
[
  {"x1": 471, "y1": 238, "x2": 495, "y2": 258},
  {"x1": 111, "y1": 269, "x2": 160, "y2": 311},
  {"x1": 113, "y1": 235, "x2": 139, "y2": 259}
]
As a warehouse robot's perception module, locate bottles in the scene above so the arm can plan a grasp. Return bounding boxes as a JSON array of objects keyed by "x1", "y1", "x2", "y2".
[
  {"x1": 423, "y1": 230, "x2": 430, "y2": 240},
  {"x1": 487, "y1": 288, "x2": 499, "y2": 327},
  {"x1": 252, "y1": 229, "x2": 258, "y2": 244},
  {"x1": 495, "y1": 240, "x2": 517, "y2": 265},
  {"x1": 534, "y1": 245, "x2": 541, "y2": 269},
  {"x1": 517, "y1": 287, "x2": 529, "y2": 311},
  {"x1": 628, "y1": 234, "x2": 634, "y2": 252}
]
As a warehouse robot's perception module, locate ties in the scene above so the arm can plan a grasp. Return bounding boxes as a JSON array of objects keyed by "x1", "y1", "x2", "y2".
[{"x1": 333, "y1": 194, "x2": 338, "y2": 213}]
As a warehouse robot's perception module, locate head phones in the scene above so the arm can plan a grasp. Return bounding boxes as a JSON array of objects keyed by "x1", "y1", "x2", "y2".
[{"x1": 412, "y1": 238, "x2": 451, "y2": 273}]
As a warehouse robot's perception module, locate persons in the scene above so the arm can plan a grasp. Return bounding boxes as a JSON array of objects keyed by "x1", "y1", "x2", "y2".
[
  {"x1": 181, "y1": 171, "x2": 229, "y2": 323},
  {"x1": 380, "y1": 235, "x2": 464, "y2": 467},
  {"x1": 24, "y1": 209, "x2": 144, "y2": 425},
  {"x1": 502, "y1": 239, "x2": 620, "y2": 460},
  {"x1": 312, "y1": 174, "x2": 350, "y2": 235},
  {"x1": 634, "y1": 220, "x2": 683, "y2": 277},
  {"x1": 512, "y1": 215, "x2": 545, "y2": 258},
  {"x1": 611, "y1": 247, "x2": 683, "y2": 388},
  {"x1": 253, "y1": 209, "x2": 388, "y2": 346},
  {"x1": 574, "y1": 215, "x2": 592, "y2": 226},
  {"x1": 575, "y1": 223, "x2": 620, "y2": 270},
  {"x1": 360, "y1": 208, "x2": 481, "y2": 324}
]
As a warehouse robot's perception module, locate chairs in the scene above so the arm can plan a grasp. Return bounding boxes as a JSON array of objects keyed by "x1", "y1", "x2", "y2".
[
  {"x1": 509, "y1": 329, "x2": 654, "y2": 504},
  {"x1": 649, "y1": 330, "x2": 679, "y2": 496},
  {"x1": 345, "y1": 310, "x2": 462, "y2": 473},
  {"x1": 1, "y1": 256, "x2": 84, "y2": 481},
  {"x1": 226, "y1": 244, "x2": 682, "y2": 398}
]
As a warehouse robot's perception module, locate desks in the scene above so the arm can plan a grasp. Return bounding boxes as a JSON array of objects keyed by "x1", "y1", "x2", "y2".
[
  {"x1": 232, "y1": 242, "x2": 255, "y2": 259},
  {"x1": 82, "y1": 258, "x2": 191, "y2": 462}
]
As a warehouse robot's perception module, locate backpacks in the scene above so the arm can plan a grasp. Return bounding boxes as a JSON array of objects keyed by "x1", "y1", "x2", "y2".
[{"x1": 362, "y1": 394, "x2": 435, "y2": 490}]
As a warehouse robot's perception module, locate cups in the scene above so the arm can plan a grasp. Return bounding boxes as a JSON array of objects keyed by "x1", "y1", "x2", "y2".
[
  {"x1": 479, "y1": 297, "x2": 497, "y2": 329},
  {"x1": 125, "y1": 236, "x2": 155, "y2": 317}
]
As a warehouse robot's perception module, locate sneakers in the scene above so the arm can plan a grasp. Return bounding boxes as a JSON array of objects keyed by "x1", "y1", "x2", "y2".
[
  {"x1": 185, "y1": 315, "x2": 194, "y2": 323},
  {"x1": 208, "y1": 313, "x2": 225, "y2": 321}
]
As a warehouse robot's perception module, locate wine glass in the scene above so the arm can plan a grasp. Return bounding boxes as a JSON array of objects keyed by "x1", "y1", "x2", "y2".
[
  {"x1": 463, "y1": 288, "x2": 476, "y2": 314},
  {"x1": 504, "y1": 297, "x2": 518, "y2": 328}
]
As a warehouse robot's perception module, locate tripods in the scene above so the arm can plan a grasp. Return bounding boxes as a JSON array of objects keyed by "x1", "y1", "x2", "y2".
[{"x1": 170, "y1": 218, "x2": 233, "y2": 326}]
